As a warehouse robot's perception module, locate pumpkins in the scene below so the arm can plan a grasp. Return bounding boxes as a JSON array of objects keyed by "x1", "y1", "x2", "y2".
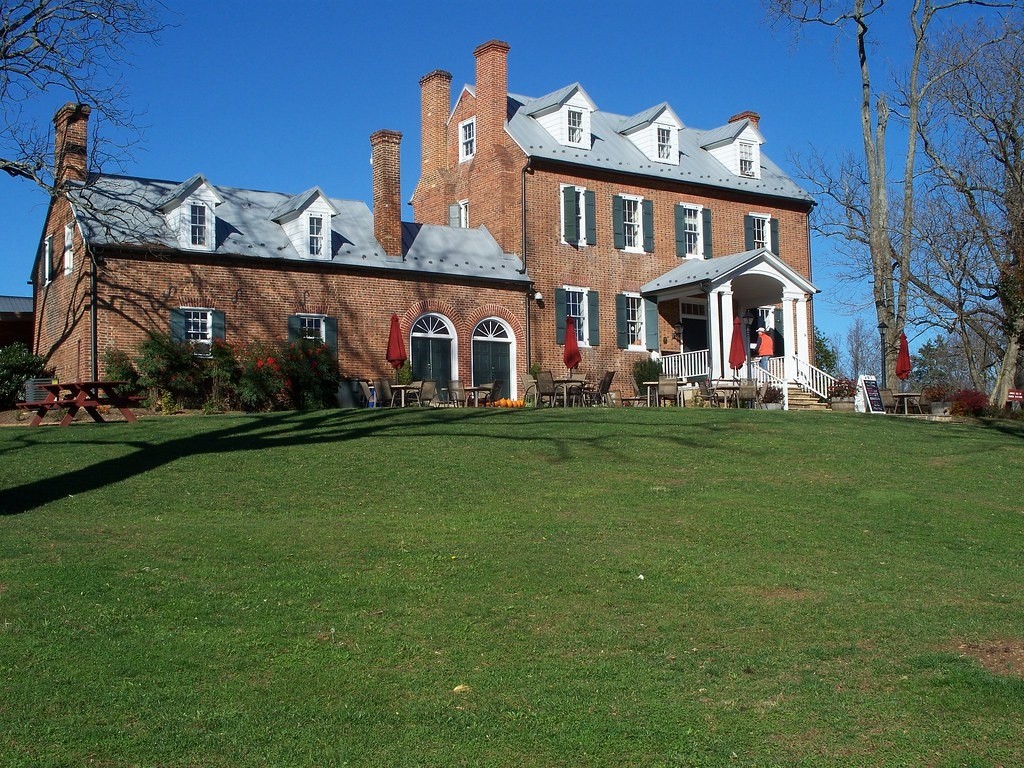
[{"x1": 486, "y1": 398, "x2": 525, "y2": 407}]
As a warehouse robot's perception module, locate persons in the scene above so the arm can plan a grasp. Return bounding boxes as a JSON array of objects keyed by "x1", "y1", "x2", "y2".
[{"x1": 753, "y1": 327, "x2": 774, "y2": 382}]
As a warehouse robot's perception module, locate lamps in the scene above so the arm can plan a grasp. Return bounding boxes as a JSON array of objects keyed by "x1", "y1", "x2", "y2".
[{"x1": 672, "y1": 321, "x2": 683, "y2": 340}]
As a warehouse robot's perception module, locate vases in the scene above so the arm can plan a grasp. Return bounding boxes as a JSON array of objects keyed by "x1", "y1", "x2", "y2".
[{"x1": 832, "y1": 397, "x2": 855, "y2": 411}]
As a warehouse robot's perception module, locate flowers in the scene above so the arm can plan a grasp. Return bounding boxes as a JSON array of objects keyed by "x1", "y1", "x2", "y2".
[{"x1": 828, "y1": 374, "x2": 860, "y2": 401}]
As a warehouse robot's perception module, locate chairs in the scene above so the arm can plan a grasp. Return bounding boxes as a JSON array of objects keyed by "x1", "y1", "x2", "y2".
[
  {"x1": 658, "y1": 374, "x2": 682, "y2": 408},
  {"x1": 697, "y1": 378, "x2": 769, "y2": 410},
  {"x1": 358, "y1": 376, "x2": 438, "y2": 408},
  {"x1": 428, "y1": 379, "x2": 497, "y2": 408},
  {"x1": 628, "y1": 375, "x2": 655, "y2": 407},
  {"x1": 880, "y1": 389, "x2": 928, "y2": 414},
  {"x1": 521, "y1": 370, "x2": 617, "y2": 408}
]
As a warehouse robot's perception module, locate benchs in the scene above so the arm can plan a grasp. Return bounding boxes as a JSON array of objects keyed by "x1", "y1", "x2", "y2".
[
  {"x1": 15, "y1": 400, "x2": 77, "y2": 407},
  {"x1": 95, "y1": 396, "x2": 148, "y2": 402}
]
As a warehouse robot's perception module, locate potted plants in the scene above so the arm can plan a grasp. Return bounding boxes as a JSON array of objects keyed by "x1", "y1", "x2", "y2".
[{"x1": 757, "y1": 386, "x2": 784, "y2": 410}]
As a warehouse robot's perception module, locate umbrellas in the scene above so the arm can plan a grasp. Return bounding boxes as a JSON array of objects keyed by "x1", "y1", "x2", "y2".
[
  {"x1": 386, "y1": 313, "x2": 407, "y2": 385},
  {"x1": 729, "y1": 317, "x2": 745, "y2": 378},
  {"x1": 563, "y1": 316, "x2": 580, "y2": 380},
  {"x1": 896, "y1": 334, "x2": 911, "y2": 393}
]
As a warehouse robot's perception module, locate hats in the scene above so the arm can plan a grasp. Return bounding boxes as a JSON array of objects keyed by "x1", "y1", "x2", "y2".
[{"x1": 756, "y1": 327, "x2": 766, "y2": 332}]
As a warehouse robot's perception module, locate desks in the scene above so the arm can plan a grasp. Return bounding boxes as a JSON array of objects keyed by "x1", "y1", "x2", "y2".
[
  {"x1": 29, "y1": 382, "x2": 139, "y2": 426},
  {"x1": 643, "y1": 382, "x2": 686, "y2": 408},
  {"x1": 891, "y1": 393, "x2": 923, "y2": 415},
  {"x1": 369, "y1": 384, "x2": 421, "y2": 408},
  {"x1": 529, "y1": 379, "x2": 590, "y2": 408},
  {"x1": 711, "y1": 379, "x2": 740, "y2": 408},
  {"x1": 441, "y1": 387, "x2": 491, "y2": 407}
]
